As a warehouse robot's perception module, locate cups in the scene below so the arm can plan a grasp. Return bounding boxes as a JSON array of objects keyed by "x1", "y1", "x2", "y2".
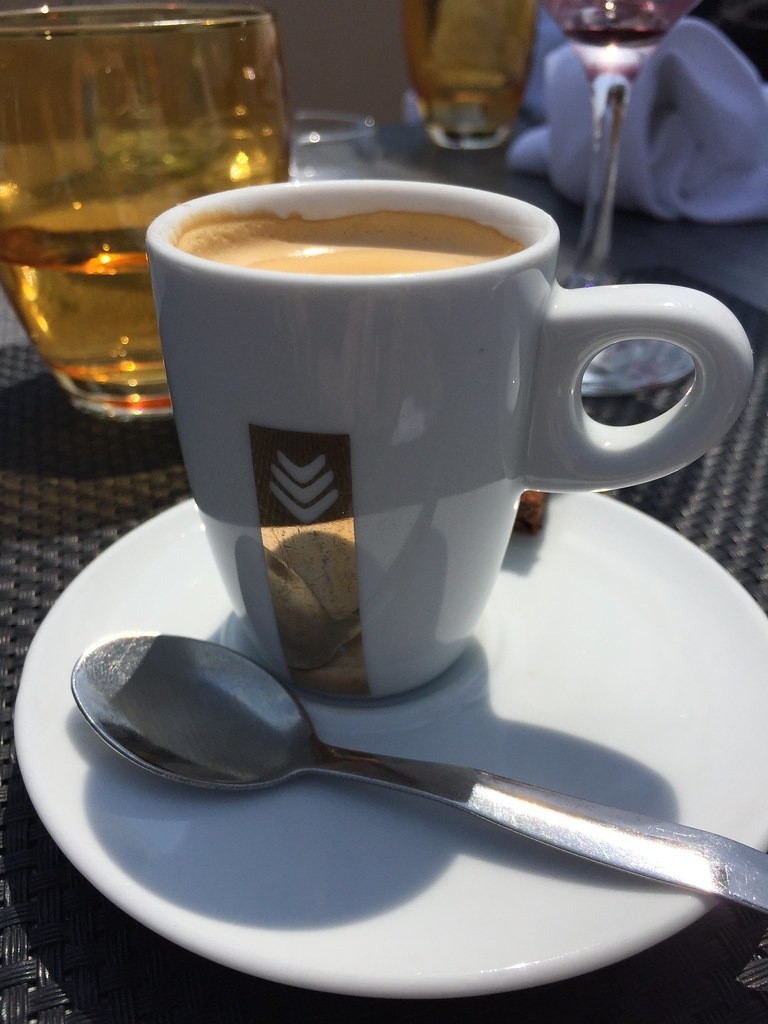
[
  {"x1": 400, "y1": 0, "x2": 537, "y2": 152},
  {"x1": 0, "y1": 0, "x2": 294, "y2": 416},
  {"x1": 193, "y1": 105, "x2": 378, "y2": 184},
  {"x1": 145, "y1": 175, "x2": 753, "y2": 705}
]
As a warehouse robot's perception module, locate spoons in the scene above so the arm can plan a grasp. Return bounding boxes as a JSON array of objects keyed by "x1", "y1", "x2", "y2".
[{"x1": 72, "y1": 635, "x2": 768, "y2": 913}]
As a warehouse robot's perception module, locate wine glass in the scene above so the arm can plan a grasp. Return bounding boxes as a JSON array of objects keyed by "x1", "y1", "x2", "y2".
[{"x1": 536, "y1": 0, "x2": 708, "y2": 285}]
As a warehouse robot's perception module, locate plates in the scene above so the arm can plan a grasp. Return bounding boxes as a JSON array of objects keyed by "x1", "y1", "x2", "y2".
[{"x1": 14, "y1": 495, "x2": 768, "y2": 1001}]
